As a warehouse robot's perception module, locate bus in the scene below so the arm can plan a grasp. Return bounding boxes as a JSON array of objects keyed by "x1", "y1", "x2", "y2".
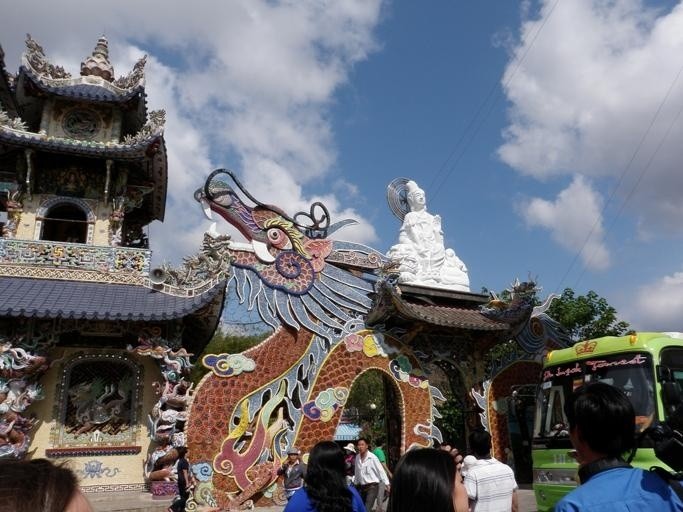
[{"x1": 531, "y1": 331, "x2": 683, "y2": 512}]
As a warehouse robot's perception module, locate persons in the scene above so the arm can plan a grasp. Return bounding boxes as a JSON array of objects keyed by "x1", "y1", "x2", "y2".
[
  {"x1": 556, "y1": 381, "x2": 683, "y2": 512},
  {"x1": 0, "y1": 458, "x2": 95, "y2": 512},
  {"x1": 384, "y1": 444, "x2": 470, "y2": 512},
  {"x1": 277, "y1": 438, "x2": 393, "y2": 512},
  {"x1": 460, "y1": 430, "x2": 519, "y2": 512},
  {"x1": 387, "y1": 181, "x2": 470, "y2": 293},
  {"x1": 168, "y1": 446, "x2": 190, "y2": 512}
]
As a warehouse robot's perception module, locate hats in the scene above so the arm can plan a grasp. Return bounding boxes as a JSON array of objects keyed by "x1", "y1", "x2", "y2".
[
  {"x1": 287, "y1": 448, "x2": 298, "y2": 455},
  {"x1": 343, "y1": 443, "x2": 357, "y2": 454}
]
